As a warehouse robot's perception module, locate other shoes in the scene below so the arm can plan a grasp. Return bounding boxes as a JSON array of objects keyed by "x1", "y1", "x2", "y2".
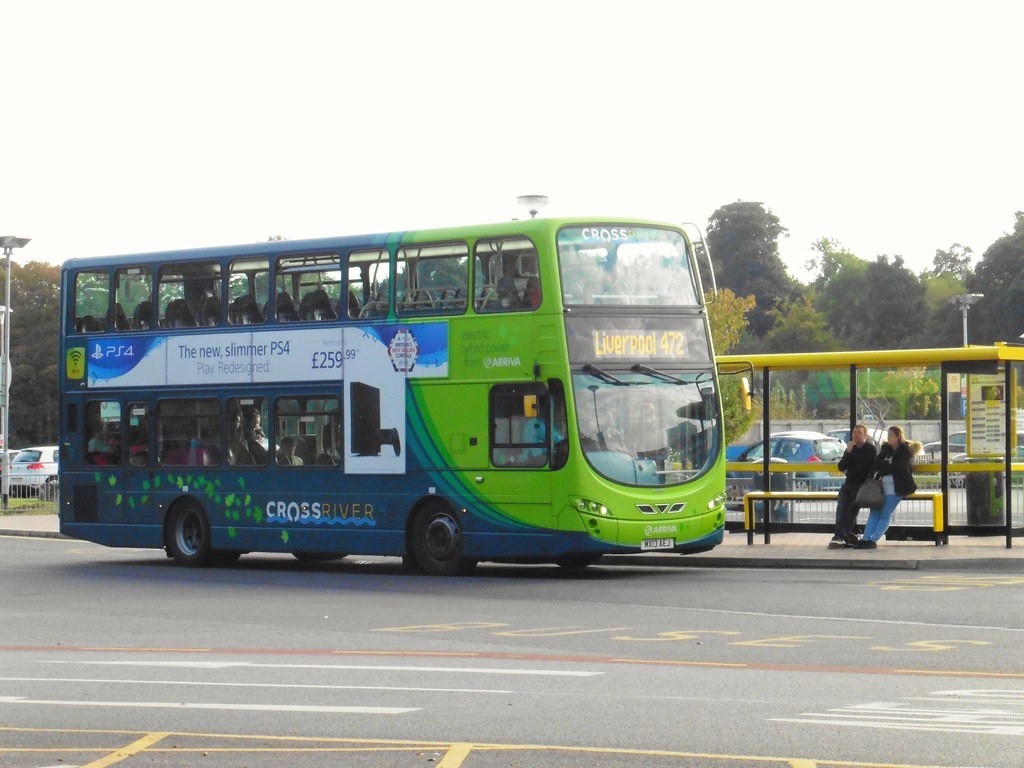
[
  {"x1": 828, "y1": 536, "x2": 847, "y2": 548},
  {"x1": 854, "y1": 539, "x2": 877, "y2": 549},
  {"x1": 844, "y1": 539, "x2": 858, "y2": 547}
]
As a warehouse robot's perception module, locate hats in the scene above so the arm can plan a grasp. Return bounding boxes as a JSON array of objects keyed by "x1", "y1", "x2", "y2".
[{"x1": 280, "y1": 437, "x2": 295, "y2": 447}]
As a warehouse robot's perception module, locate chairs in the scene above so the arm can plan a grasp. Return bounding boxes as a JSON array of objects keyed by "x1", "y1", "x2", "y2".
[
  {"x1": 77, "y1": 290, "x2": 498, "y2": 333},
  {"x1": 494, "y1": 416, "x2": 528, "y2": 465}
]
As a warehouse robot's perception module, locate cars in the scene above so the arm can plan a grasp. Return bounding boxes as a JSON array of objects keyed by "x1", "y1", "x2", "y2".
[
  {"x1": 0, "y1": 445, "x2": 60, "y2": 504},
  {"x1": 85, "y1": 428, "x2": 222, "y2": 467},
  {"x1": 724, "y1": 430, "x2": 850, "y2": 501},
  {"x1": 826, "y1": 423, "x2": 927, "y2": 464},
  {"x1": 922, "y1": 428, "x2": 1024, "y2": 489}
]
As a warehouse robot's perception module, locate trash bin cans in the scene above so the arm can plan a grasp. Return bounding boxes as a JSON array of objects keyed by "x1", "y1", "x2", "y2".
[
  {"x1": 964, "y1": 457, "x2": 1004, "y2": 536},
  {"x1": 753, "y1": 457, "x2": 789, "y2": 523}
]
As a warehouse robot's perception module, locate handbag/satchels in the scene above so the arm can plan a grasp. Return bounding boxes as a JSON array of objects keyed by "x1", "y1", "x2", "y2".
[{"x1": 852, "y1": 465, "x2": 886, "y2": 509}]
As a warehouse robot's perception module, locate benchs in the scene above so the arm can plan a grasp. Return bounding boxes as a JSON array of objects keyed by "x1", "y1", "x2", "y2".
[{"x1": 743, "y1": 491, "x2": 945, "y2": 546}]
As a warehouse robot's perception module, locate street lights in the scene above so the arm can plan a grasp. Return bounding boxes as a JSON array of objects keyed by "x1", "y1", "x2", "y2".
[
  {"x1": 586, "y1": 384, "x2": 608, "y2": 451},
  {"x1": 947, "y1": 292, "x2": 985, "y2": 347},
  {"x1": 0, "y1": 234, "x2": 33, "y2": 509},
  {"x1": 0, "y1": 306, "x2": 14, "y2": 449}
]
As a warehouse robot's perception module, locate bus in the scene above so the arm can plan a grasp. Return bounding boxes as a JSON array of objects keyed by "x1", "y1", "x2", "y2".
[{"x1": 53, "y1": 216, "x2": 757, "y2": 577}]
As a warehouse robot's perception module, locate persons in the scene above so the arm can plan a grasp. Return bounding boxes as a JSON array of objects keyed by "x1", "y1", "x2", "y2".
[
  {"x1": 631, "y1": 403, "x2": 668, "y2": 451},
  {"x1": 496, "y1": 265, "x2": 541, "y2": 309},
  {"x1": 88, "y1": 418, "x2": 119, "y2": 455},
  {"x1": 829, "y1": 425, "x2": 877, "y2": 548},
  {"x1": 276, "y1": 437, "x2": 304, "y2": 465},
  {"x1": 854, "y1": 426, "x2": 922, "y2": 549},
  {"x1": 224, "y1": 410, "x2": 258, "y2": 465},
  {"x1": 520, "y1": 410, "x2": 564, "y2": 460},
  {"x1": 242, "y1": 406, "x2": 280, "y2": 462}
]
